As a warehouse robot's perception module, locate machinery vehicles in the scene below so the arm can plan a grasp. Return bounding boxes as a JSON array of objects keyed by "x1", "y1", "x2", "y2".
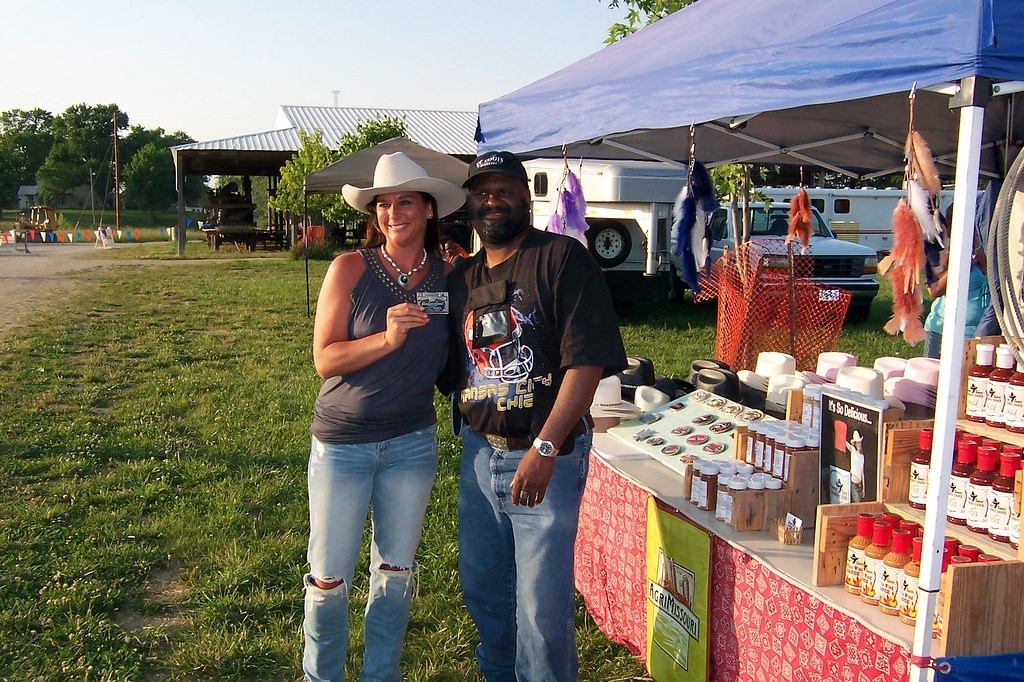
[{"x1": 202, "y1": 180, "x2": 258, "y2": 251}]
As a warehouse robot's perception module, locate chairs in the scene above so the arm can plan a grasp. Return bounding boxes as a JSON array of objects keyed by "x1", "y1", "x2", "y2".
[{"x1": 769, "y1": 218, "x2": 789, "y2": 235}]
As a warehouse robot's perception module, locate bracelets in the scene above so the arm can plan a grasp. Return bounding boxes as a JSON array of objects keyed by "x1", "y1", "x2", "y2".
[{"x1": 975, "y1": 246, "x2": 980, "y2": 251}]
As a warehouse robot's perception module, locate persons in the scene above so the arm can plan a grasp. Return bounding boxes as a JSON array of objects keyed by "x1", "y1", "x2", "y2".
[
  {"x1": 846, "y1": 430, "x2": 865, "y2": 502},
  {"x1": 923, "y1": 202, "x2": 1003, "y2": 359},
  {"x1": 303, "y1": 151, "x2": 455, "y2": 682},
  {"x1": 443, "y1": 151, "x2": 629, "y2": 682}
]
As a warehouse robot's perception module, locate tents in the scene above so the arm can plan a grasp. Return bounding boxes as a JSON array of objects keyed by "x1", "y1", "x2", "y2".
[{"x1": 475, "y1": 0, "x2": 1024, "y2": 681}]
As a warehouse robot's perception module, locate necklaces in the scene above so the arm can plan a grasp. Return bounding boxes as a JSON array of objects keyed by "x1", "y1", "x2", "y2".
[{"x1": 380, "y1": 244, "x2": 427, "y2": 287}]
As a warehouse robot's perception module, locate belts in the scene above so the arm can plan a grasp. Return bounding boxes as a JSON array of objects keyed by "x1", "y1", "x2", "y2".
[{"x1": 479, "y1": 413, "x2": 595, "y2": 452}]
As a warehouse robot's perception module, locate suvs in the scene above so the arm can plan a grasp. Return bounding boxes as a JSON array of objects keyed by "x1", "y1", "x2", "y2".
[{"x1": 702, "y1": 200, "x2": 880, "y2": 322}]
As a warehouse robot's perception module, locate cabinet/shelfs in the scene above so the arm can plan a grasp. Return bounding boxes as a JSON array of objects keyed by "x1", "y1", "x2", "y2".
[
  {"x1": 811, "y1": 334, "x2": 1024, "y2": 659},
  {"x1": 682, "y1": 389, "x2": 819, "y2": 533}
]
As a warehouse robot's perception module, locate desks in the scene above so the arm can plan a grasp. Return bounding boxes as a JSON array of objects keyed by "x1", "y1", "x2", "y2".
[{"x1": 571, "y1": 433, "x2": 1024, "y2": 682}]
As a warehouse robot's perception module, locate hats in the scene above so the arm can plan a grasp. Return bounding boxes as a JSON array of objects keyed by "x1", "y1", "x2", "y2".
[
  {"x1": 342, "y1": 151, "x2": 467, "y2": 219},
  {"x1": 588, "y1": 351, "x2": 944, "y2": 422},
  {"x1": 461, "y1": 151, "x2": 529, "y2": 189}
]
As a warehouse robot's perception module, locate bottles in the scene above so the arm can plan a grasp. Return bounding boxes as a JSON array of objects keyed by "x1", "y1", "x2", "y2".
[
  {"x1": 689, "y1": 382, "x2": 889, "y2": 527},
  {"x1": 841, "y1": 343, "x2": 1024, "y2": 647}
]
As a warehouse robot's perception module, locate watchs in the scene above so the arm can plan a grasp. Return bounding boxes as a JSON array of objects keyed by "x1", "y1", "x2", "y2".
[{"x1": 533, "y1": 438, "x2": 559, "y2": 458}]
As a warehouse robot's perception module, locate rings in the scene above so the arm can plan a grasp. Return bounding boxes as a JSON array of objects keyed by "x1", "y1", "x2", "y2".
[{"x1": 520, "y1": 490, "x2": 528, "y2": 499}]
